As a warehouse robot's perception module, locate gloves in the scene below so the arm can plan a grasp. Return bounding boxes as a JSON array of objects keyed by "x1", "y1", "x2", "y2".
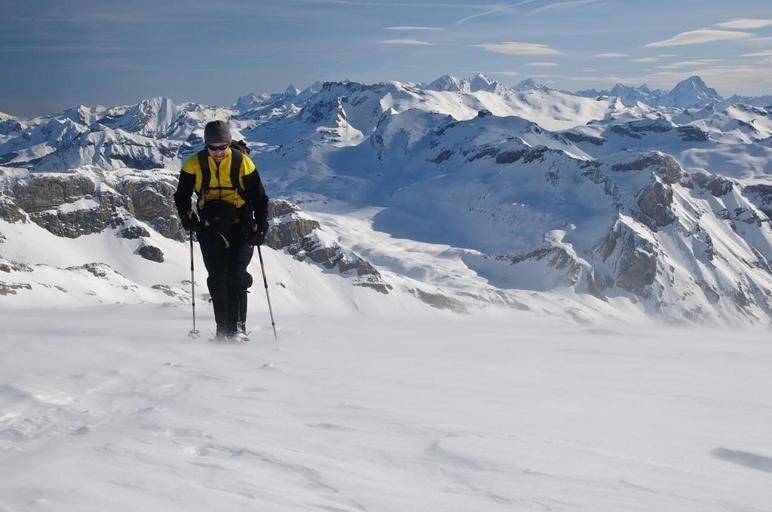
[
  {"x1": 250, "y1": 226, "x2": 265, "y2": 246},
  {"x1": 182, "y1": 218, "x2": 200, "y2": 232}
]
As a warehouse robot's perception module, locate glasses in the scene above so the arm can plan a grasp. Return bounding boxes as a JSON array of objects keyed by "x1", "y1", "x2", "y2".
[{"x1": 207, "y1": 143, "x2": 229, "y2": 151}]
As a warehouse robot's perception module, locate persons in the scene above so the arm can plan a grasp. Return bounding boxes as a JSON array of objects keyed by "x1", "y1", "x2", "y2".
[{"x1": 175, "y1": 122, "x2": 269, "y2": 343}]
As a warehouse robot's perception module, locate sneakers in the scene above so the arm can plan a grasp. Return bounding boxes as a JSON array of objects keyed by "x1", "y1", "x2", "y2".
[{"x1": 215, "y1": 322, "x2": 249, "y2": 344}]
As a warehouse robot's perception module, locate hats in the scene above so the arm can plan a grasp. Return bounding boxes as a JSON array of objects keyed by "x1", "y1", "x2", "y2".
[{"x1": 204, "y1": 120, "x2": 232, "y2": 144}]
